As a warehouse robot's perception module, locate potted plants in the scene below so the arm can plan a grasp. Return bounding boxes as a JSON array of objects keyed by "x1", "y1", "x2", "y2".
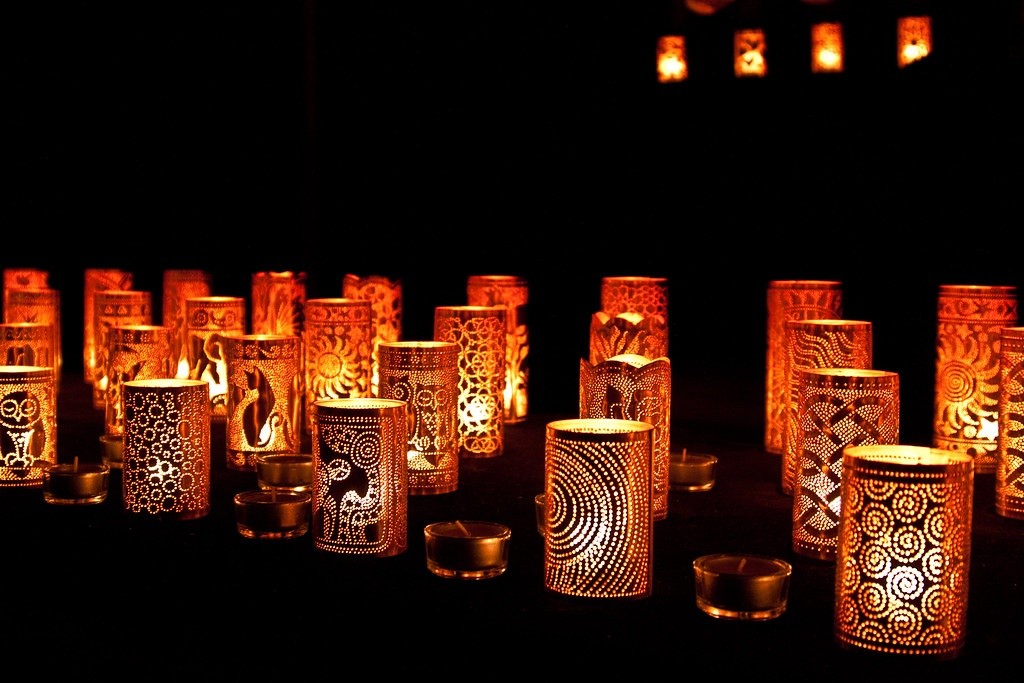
[{"x1": 99, "y1": 431, "x2": 123, "y2": 467}]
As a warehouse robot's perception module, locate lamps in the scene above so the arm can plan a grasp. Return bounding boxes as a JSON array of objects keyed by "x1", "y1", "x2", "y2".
[
  {"x1": 590, "y1": 311, "x2": 670, "y2": 364},
  {"x1": 780, "y1": 319, "x2": 873, "y2": 494},
  {"x1": 734, "y1": 29, "x2": 766, "y2": 76},
  {"x1": 996, "y1": 324, "x2": 1024, "y2": 517},
  {"x1": 937, "y1": 284, "x2": 1016, "y2": 473},
  {"x1": 897, "y1": 17, "x2": 931, "y2": 68},
  {"x1": 657, "y1": 35, "x2": 689, "y2": 81},
  {"x1": 830, "y1": 444, "x2": 974, "y2": 653},
  {"x1": 0, "y1": 271, "x2": 530, "y2": 558},
  {"x1": 792, "y1": 368, "x2": 900, "y2": 561},
  {"x1": 602, "y1": 276, "x2": 670, "y2": 312},
  {"x1": 546, "y1": 418, "x2": 654, "y2": 600},
  {"x1": 580, "y1": 353, "x2": 669, "y2": 520},
  {"x1": 764, "y1": 279, "x2": 842, "y2": 455},
  {"x1": 812, "y1": 23, "x2": 842, "y2": 71}
]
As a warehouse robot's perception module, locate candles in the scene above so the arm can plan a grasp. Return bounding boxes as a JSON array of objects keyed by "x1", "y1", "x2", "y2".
[
  {"x1": 256, "y1": 451, "x2": 314, "y2": 493},
  {"x1": 235, "y1": 488, "x2": 312, "y2": 538},
  {"x1": 692, "y1": 554, "x2": 792, "y2": 622},
  {"x1": 424, "y1": 520, "x2": 512, "y2": 580},
  {"x1": 670, "y1": 448, "x2": 718, "y2": 491},
  {"x1": 42, "y1": 457, "x2": 110, "y2": 505}
]
